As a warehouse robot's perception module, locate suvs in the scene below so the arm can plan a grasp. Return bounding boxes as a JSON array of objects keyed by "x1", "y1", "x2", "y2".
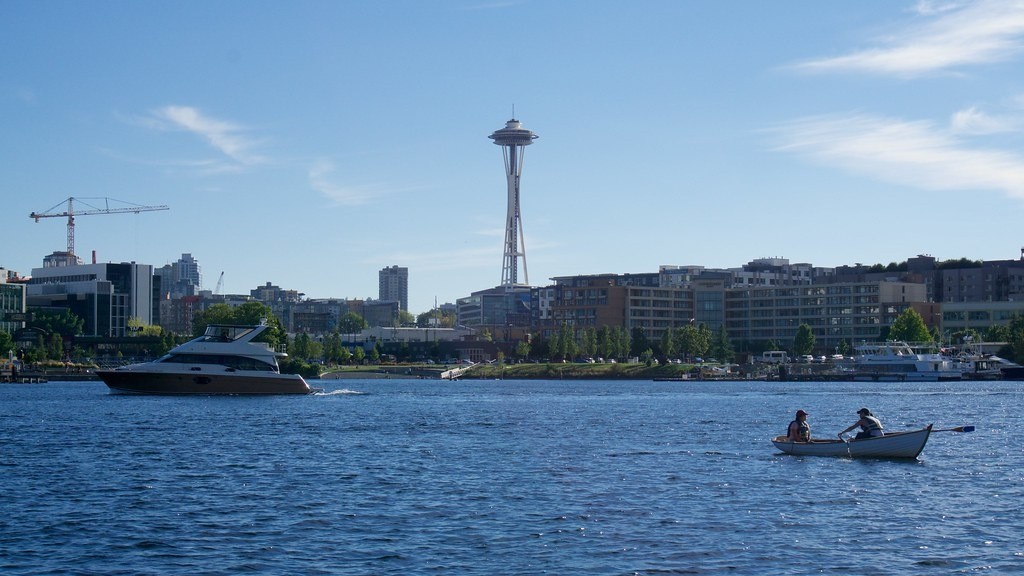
[{"x1": 798, "y1": 355, "x2": 813, "y2": 363}]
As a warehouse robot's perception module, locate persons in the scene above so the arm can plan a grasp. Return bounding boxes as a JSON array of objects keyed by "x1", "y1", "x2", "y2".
[
  {"x1": 222, "y1": 330, "x2": 228, "y2": 337},
  {"x1": 787, "y1": 410, "x2": 811, "y2": 442},
  {"x1": 838, "y1": 407, "x2": 884, "y2": 439}
]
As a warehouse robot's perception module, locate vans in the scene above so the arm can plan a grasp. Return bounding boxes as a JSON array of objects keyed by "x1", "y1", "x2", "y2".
[{"x1": 827, "y1": 354, "x2": 843, "y2": 361}]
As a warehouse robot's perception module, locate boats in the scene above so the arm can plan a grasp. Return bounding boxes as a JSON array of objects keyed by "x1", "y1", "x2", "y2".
[
  {"x1": 92, "y1": 323, "x2": 315, "y2": 395},
  {"x1": 843, "y1": 339, "x2": 962, "y2": 382},
  {"x1": 939, "y1": 332, "x2": 1024, "y2": 381},
  {"x1": 774, "y1": 421, "x2": 932, "y2": 462}
]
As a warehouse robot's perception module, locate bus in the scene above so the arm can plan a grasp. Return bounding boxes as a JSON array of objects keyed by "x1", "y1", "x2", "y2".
[{"x1": 762, "y1": 351, "x2": 791, "y2": 364}]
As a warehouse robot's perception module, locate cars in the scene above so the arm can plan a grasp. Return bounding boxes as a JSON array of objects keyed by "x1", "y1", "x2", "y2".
[
  {"x1": 423, "y1": 356, "x2": 605, "y2": 364},
  {"x1": 815, "y1": 356, "x2": 826, "y2": 363},
  {"x1": 694, "y1": 366, "x2": 726, "y2": 373},
  {"x1": 692, "y1": 357, "x2": 718, "y2": 363},
  {"x1": 672, "y1": 359, "x2": 681, "y2": 364},
  {"x1": 606, "y1": 359, "x2": 616, "y2": 363}
]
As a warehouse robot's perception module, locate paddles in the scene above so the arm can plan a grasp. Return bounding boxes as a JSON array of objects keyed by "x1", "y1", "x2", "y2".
[
  {"x1": 884, "y1": 426, "x2": 975, "y2": 435},
  {"x1": 837, "y1": 434, "x2": 852, "y2": 454}
]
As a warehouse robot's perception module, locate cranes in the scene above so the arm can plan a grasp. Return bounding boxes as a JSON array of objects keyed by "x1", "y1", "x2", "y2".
[
  {"x1": 212, "y1": 271, "x2": 224, "y2": 300},
  {"x1": 28, "y1": 195, "x2": 169, "y2": 264}
]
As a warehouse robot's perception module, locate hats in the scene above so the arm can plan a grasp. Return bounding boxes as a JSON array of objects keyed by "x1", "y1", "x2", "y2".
[
  {"x1": 857, "y1": 408, "x2": 869, "y2": 413},
  {"x1": 796, "y1": 410, "x2": 808, "y2": 416}
]
